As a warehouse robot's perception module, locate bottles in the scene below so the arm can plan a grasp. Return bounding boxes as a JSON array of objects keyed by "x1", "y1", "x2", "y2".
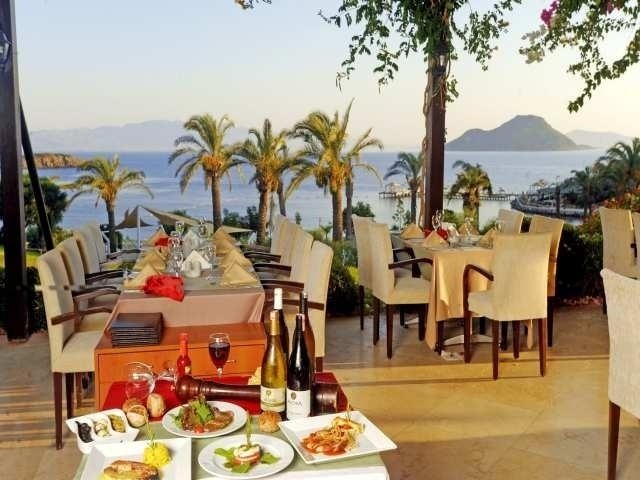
[
  {"x1": 175, "y1": 333, "x2": 194, "y2": 380},
  {"x1": 260, "y1": 285, "x2": 315, "y2": 419}
]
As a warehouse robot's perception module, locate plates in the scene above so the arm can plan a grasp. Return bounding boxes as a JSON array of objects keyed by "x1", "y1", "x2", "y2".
[
  {"x1": 66, "y1": 411, "x2": 139, "y2": 451},
  {"x1": 275, "y1": 408, "x2": 397, "y2": 466},
  {"x1": 196, "y1": 433, "x2": 293, "y2": 479},
  {"x1": 77, "y1": 437, "x2": 193, "y2": 480},
  {"x1": 158, "y1": 399, "x2": 250, "y2": 439}
]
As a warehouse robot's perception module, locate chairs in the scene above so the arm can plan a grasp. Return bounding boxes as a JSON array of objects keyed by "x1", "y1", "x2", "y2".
[
  {"x1": 365, "y1": 221, "x2": 434, "y2": 361},
  {"x1": 461, "y1": 233, "x2": 554, "y2": 380},
  {"x1": 491, "y1": 208, "x2": 526, "y2": 237},
  {"x1": 351, "y1": 214, "x2": 376, "y2": 329},
  {"x1": 477, "y1": 214, "x2": 564, "y2": 350},
  {"x1": 597, "y1": 204, "x2": 640, "y2": 479}
]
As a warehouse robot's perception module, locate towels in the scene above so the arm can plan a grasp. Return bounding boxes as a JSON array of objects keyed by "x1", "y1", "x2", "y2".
[
  {"x1": 479, "y1": 228, "x2": 494, "y2": 244},
  {"x1": 456, "y1": 224, "x2": 471, "y2": 234},
  {"x1": 401, "y1": 222, "x2": 423, "y2": 240},
  {"x1": 423, "y1": 230, "x2": 447, "y2": 247}
]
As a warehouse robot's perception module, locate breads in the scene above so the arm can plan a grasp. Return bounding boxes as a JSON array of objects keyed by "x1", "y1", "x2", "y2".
[
  {"x1": 103, "y1": 460, "x2": 159, "y2": 480},
  {"x1": 259, "y1": 411, "x2": 282, "y2": 433}
]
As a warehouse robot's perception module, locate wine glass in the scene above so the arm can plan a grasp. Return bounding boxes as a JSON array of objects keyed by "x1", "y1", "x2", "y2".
[
  {"x1": 198, "y1": 215, "x2": 221, "y2": 283},
  {"x1": 123, "y1": 361, "x2": 177, "y2": 399},
  {"x1": 168, "y1": 218, "x2": 184, "y2": 280},
  {"x1": 431, "y1": 207, "x2": 504, "y2": 249},
  {"x1": 205, "y1": 332, "x2": 234, "y2": 381}
]
